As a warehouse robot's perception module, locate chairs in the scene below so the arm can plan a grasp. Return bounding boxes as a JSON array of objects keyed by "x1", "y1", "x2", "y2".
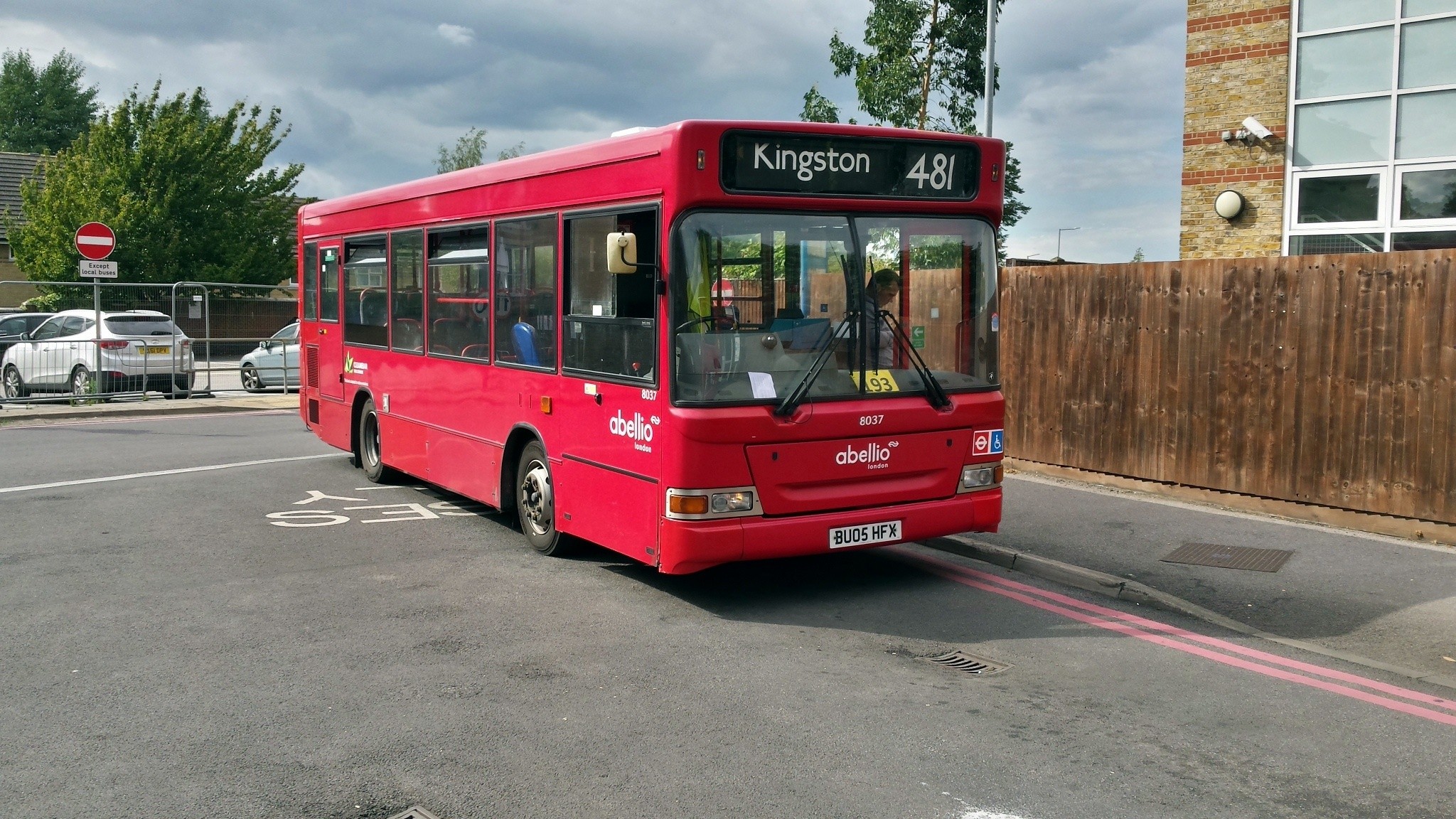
[
  {"x1": 311, "y1": 286, "x2": 553, "y2": 366},
  {"x1": 11, "y1": 321, "x2": 25, "y2": 335}
]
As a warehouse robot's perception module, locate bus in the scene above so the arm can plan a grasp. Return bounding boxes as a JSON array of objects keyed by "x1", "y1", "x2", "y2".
[{"x1": 296, "y1": 118, "x2": 1008, "y2": 576}]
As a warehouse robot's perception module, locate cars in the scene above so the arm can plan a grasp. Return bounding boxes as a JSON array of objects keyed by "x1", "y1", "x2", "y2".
[
  {"x1": 0, "y1": 312, "x2": 58, "y2": 367},
  {"x1": 238, "y1": 322, "x2": 302, "y2": 393}
]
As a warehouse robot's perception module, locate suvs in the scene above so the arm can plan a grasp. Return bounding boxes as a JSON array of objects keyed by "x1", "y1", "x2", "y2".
[{"x1": 1, "y1": 307, "x2": 197, "y2": 403}]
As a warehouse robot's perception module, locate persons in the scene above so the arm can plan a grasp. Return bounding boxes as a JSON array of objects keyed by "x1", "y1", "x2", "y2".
[{"x1": 853, "y1": 268, "x2": 899, "y2": 370}]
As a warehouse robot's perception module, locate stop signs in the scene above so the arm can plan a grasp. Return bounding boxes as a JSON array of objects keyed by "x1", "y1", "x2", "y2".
[
  {"x1": 74, "y1": 221, "x2": 115, "y2": 260},
  {"x1": 710, "y1": 278, "x2": 734, "y2": 307}
]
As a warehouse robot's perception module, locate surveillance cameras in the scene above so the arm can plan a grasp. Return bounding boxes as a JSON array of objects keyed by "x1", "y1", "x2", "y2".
[{"x1": 1241, "y1": 116, "x2": 1274, "y2": 139}]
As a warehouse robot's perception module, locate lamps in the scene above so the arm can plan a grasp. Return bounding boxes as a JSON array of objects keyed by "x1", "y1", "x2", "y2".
[{"x1": 1214, "y1": 190, "x2": 1245, "y2": 220}]
